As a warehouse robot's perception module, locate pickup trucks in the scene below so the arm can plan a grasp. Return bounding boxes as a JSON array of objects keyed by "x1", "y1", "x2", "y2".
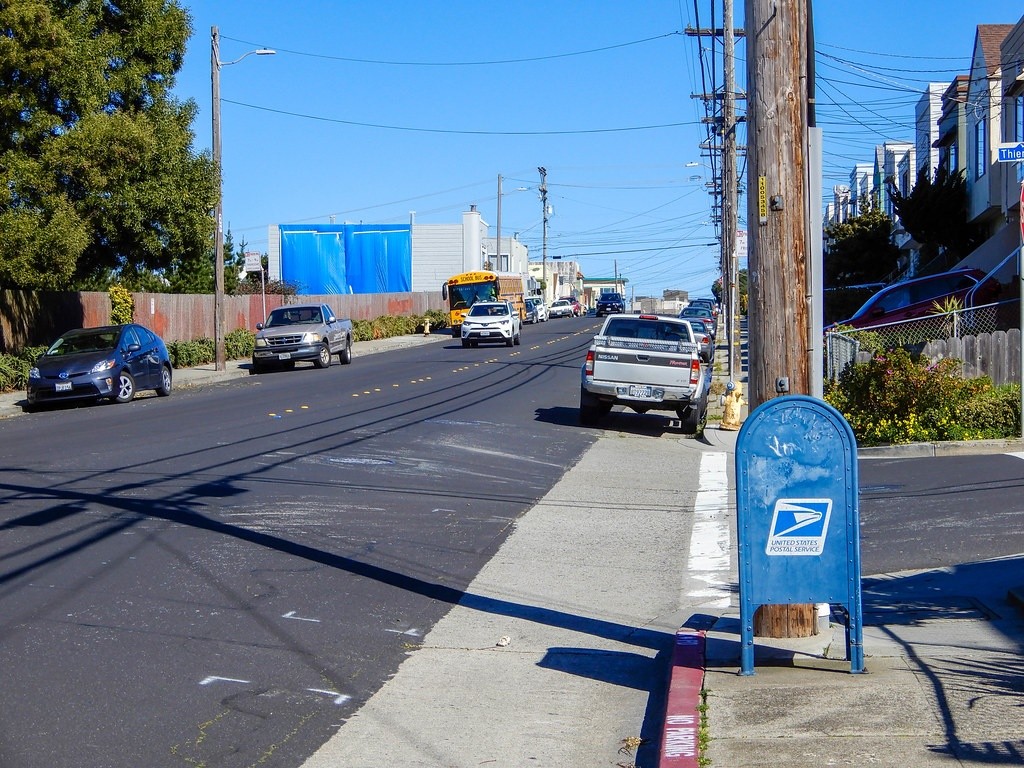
[
  {"x1": 254, "y1": 303, "x2": 354, "y2": 370},
  {"x1": 578, "y1": 314, "x2": 709, "y2": 434}
]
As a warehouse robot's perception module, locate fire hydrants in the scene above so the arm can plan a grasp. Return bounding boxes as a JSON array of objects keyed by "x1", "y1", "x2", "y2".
[
  {"x1": 719, "y1": 381, "x2": 747, "y2": 431},
  {"x1": 423, "y1": 318, "x2": 431, "y2": 337}
]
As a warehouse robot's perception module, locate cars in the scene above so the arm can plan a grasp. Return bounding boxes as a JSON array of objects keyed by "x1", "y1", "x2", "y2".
[
  {"x1": 24, "y1": 324, "x2": 173, "y2": 410},
  {"x1": 678, "y1": 307, "x2": 718, "y2": 337},
  {"x1": 687, "y1": 298, "x2": 721, "y2": 318},
  {"x1": 823, "y1": 265, "x2": 1010, "y2": 352},
  {"x1": 667, "y1": 317, "x2": 715, "y2": 363},
  {"x1": 548, "y1": 300, "x2": 575, "y2": 318},
  {"x1": 524, "y1": 300, "x2": 539, "y2": 324},
  {"x1": 529, "y1": 297, "x2": 549, "y2": 322}
]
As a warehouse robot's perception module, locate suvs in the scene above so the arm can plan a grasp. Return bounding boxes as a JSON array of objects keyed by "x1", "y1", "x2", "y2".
[
  {"x1": 460, "y1": 300, "x2": 523, "y2": 347},
  {"x1": 594, "y1": 293, "x2": 626, "y2": 317},
  {"x1": 559, "y1": 296, "x2": 583, "y2": 317}
]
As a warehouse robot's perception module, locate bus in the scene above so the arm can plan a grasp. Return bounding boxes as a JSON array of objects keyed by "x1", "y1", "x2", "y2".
[{"x1": 442, "y1": 271, "x2": 527, "y2": 332}]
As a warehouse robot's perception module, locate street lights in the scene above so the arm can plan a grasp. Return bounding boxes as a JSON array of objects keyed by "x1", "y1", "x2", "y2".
[
  {"x1": 209, "y1": 25, "x2": 277, "y2": 373},
  {"x1": 497, "y1": 174, "x2": 530, "y2": 272}
]
